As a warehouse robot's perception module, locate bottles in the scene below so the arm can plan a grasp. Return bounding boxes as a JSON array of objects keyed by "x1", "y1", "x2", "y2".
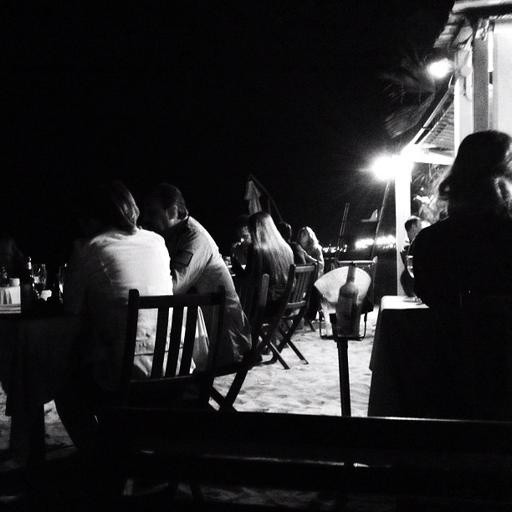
[
  {"x1": 0, "y1": 266, "x2": 10, "y2": 287},
  {"x1": 22, "y1": 255, "x2": 49, "y2": 293},
  {"x1": 336, "y1": 265, "x2": 358, "y2": 333}
]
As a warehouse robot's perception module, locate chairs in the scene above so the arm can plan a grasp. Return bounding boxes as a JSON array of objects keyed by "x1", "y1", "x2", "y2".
[{"x1": 1, "y1": 242, "x2": 379, "y2": 511}]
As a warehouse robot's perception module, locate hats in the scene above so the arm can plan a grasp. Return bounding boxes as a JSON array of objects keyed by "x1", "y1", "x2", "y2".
[{"x1": 438, "y1": 130, "x2": 512, "y2": 200}]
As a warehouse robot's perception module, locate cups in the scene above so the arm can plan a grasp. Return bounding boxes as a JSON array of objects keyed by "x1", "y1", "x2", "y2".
[{"x1": 9, "y1": 278, "x2": 20, "y2": 286}]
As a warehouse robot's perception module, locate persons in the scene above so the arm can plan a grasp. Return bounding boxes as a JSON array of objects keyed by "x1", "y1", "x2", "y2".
[
  {"x1": 0, "y1": 173, "x2": 325, "y2": 491},
  {"x1": 400, "y1": 146, "x2": 511, "y2": 423}
]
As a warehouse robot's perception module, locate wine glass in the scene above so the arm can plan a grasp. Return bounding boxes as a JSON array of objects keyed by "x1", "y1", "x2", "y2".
[{"x1": 404, "y1": 254, "x2": 426, "y2": 306}]
